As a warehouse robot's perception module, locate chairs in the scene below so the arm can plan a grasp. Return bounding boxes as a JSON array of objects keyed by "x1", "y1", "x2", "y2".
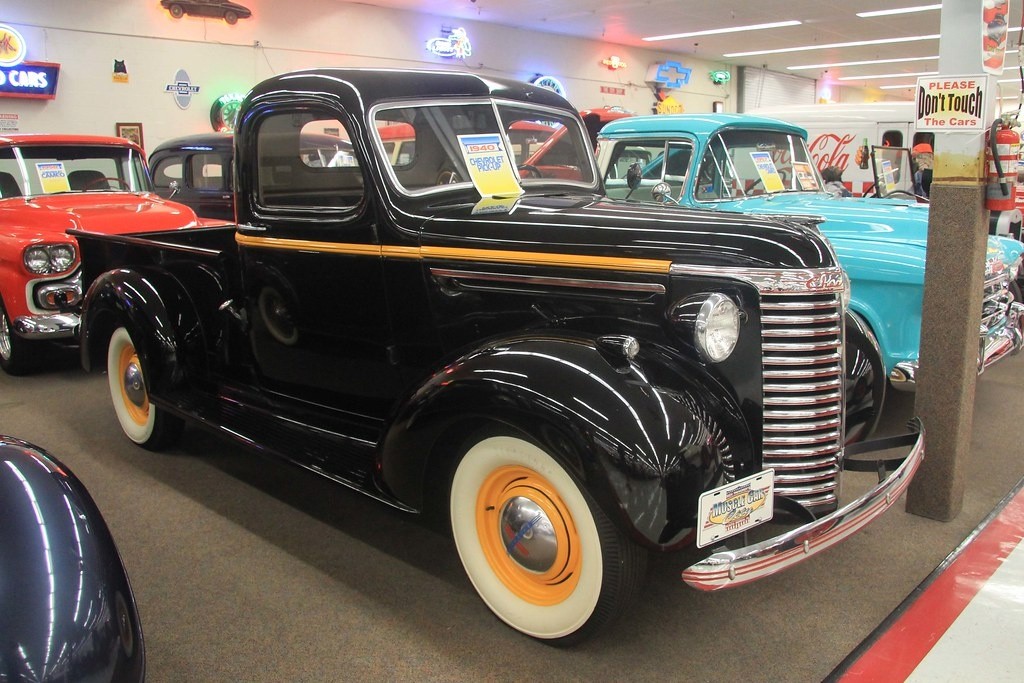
[
  {"x1": 69, "y1": 170, "x2": 110, "y2": 190},
  {"x1": 0, "y1": 172, "x2": 22, "y2": 198}
]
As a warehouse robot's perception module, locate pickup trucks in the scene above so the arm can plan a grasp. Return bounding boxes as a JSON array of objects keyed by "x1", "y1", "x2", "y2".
[{"x1": 61, "y1": 66, "x2": 927, "y2": 648}]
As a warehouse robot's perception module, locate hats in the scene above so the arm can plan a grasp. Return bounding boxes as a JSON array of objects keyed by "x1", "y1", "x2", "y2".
[{"x1": 910, "y1": 143, "x2": 932, "y2": 156}]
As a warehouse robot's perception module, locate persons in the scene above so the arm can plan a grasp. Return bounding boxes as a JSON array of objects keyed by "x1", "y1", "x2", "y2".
[
  {"x1": 913, "y1": 143, "x2": 935, "y2": 198},
  {"x1": 822, "y1": 166, "x2": 849, "y2": 197}
]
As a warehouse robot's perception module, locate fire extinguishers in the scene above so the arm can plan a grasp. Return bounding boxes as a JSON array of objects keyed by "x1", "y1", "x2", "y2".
[{"x1": 985, "y1": 107, "x2": 1022, "y2": 212}]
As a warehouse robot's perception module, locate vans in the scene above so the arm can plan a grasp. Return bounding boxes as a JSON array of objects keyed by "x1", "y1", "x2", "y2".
[{"x1": 728, "y1": 99, "x2": 935, "y2": 203}]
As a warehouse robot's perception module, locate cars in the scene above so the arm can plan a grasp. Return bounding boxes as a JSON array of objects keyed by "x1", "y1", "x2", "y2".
[
  {"x1": 516, "y1": 106, "x2": 694, "y2": 206},
  {"x1": 376, "y1": 120, "x2": 557, "y2": 167},
  {"x1": 594, "y1": 110, "x2": 1024, "y2": 395},
  {"x1": 0, "y1": 132, "x2": 236, "y2": 376},
  {"x1": 148, "y1": 127, "x2": 365, "y2": 226}
]
